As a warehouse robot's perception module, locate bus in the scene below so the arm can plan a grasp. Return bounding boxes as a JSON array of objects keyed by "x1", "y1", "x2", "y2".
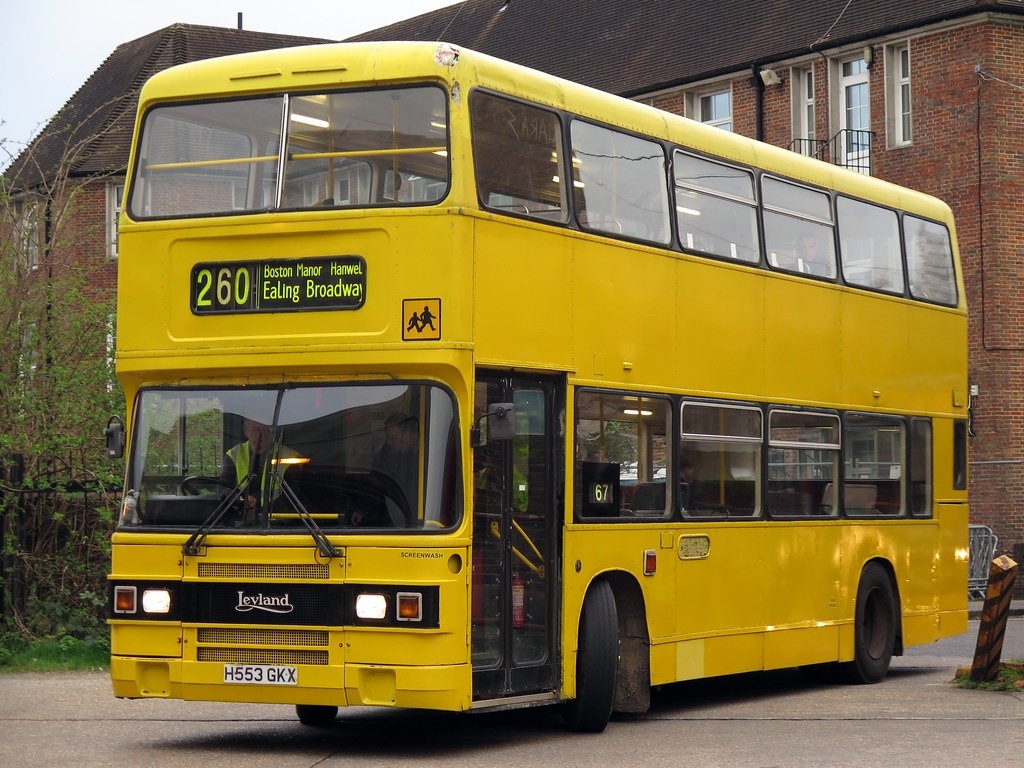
[{"x1": 106, "y1": 42, "x2": 971, "y2": 734}]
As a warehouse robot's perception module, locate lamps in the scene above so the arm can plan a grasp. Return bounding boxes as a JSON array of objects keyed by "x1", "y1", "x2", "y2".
[
  {"x1": 863, "y1": 45, "x2": 876, "y2": 69},
  {"x1": 760, "y1": 69, "x2": 785, "y2": 86}
]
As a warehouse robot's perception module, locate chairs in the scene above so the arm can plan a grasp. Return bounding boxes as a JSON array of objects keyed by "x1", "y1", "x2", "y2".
[{"x1": 624, "y1": 479, "x2": 920, "y2": 517}]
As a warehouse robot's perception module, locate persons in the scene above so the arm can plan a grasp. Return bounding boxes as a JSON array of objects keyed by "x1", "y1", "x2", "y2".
[
  {"x1": 678, "y1": 463, "x2": 697, "y2": 510},
  {"x1": 218, "y1": 410, "x2": 305, "y2": 525},
  {"x1": 794, "y1": 234, "x2": 833, "y2": 279},
  {"x1": 348, "y1": 413, "x2": 456, "y2": 528}
]
